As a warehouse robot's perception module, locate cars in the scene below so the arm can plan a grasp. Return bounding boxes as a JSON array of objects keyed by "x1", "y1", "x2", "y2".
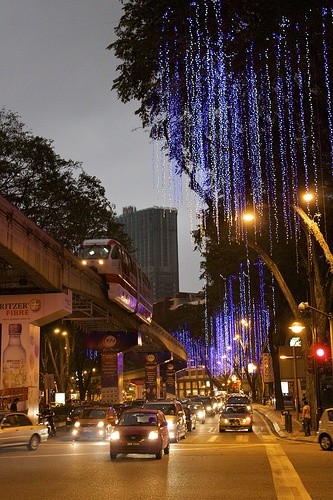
[
  {"x1": 0, "y1": 411, "x2": 49, "y2": 450},
  {"x1": 66, "y1": 393, "x2": 253, "y2": 443},
  {"x1": 109, "y1": 408, "x2": 170, "y2": 459},
  {"x1": 318, "y1": 408, "x2": 333, "y2": 450}
]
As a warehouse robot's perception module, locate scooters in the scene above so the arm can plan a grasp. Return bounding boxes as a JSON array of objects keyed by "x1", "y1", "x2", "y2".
[{"x1": 36, "y1": 414, "x2": 57, "y2": 436}]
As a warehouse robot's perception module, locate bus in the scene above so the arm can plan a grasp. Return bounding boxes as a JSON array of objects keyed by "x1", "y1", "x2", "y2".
[{"x1": 77, "y1": 238, "x2": 154, "y2": 306}]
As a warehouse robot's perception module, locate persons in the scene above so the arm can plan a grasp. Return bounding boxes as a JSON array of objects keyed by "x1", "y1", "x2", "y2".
[
  {"x1": 0, "y1": 398, "x2": 9, "y2": 410},
  {"x1": 9, "y1": 398, "x2": 19, "y2": 412},
  {"x1": 301, "y1": 400, "x2": 311, "y2": 436},
  {"x1": 42, "y1": 404, "x2": 56, "y2": 436}
]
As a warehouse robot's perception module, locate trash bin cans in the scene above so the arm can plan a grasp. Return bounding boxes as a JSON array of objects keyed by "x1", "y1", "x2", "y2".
[
  {"x1": 262, "y1": 398, "x2": 266, "y2": 406},
  {"x1": 281, "y1": 410, "x2": 294, "y2": 429}
]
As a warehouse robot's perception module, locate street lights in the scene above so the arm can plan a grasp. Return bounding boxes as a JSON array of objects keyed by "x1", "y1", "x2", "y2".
[{"x1": 289, "y1": 301, "x2": 333, "y2": 363}]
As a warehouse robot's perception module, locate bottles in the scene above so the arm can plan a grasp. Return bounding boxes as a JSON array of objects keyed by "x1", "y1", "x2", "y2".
[{"x1": 4, "y1": 324, "x2": 26, "y2": 387}]
